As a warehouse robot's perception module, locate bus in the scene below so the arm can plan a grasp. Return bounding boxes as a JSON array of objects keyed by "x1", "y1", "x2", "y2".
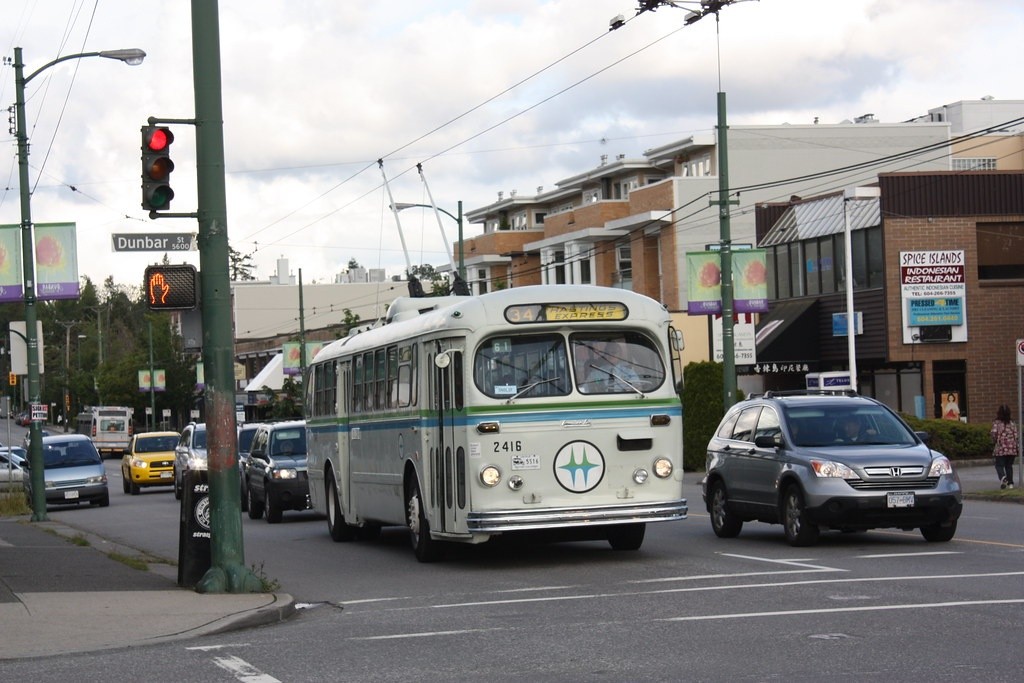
[
  {"x1": 305, "y1": 283, "x2": 685, "y2": 564},
  {"x1": 75, "y1": 406, "x2": 134, "y2": 454}
]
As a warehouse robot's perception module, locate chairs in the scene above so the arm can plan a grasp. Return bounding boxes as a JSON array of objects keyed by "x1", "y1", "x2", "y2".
[{"x1": 46, "y1": 447, "x2": 89, "y2": 464}]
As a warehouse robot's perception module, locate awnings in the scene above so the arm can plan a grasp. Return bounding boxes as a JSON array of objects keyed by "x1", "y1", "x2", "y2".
[
  {"x1": 733, "y1": 300, "x2": 821, "y2": 376},
  {"x1": 243, "y1": 352, "x2": 302, "y2": 391}
]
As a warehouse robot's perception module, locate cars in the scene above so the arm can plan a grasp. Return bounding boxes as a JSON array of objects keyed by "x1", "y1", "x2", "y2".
[
  {"x1": 0, "y1": 443, "x2": 25, "y2": 494},
  {"x1": 14, "y1": 410, "x2": 30, "y2": 427}
]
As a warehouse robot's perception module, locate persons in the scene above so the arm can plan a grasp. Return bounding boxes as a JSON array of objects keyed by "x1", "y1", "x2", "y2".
[
  {"x1": 835, "y1": 414, "x2": 883, "y2": 444},
  {"x1": 991, "y1": 405, "x2": 1022, "y2": 490},
  {"x1": 165, "y1": 439, "x2": 176, "y2": 451},
  {"x1": 279, "y1": 440, "x2": 294, "y2": 455},
  {"x1": 503, "y1": 339, "x2": 641, "y2": 398}
]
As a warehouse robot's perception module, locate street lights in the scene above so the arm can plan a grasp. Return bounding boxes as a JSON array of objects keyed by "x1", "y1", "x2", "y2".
[
  {"x1": 78, "y1": 334, "x2": 86, "y2": 414},
  {"x1": 842, "y1": 187, "x2": 882, "y2": 394},
  {"x1": 16, "y1": 48, "x2": 147, "y2": 516},
  {"x1": 390, "y1": 202, "x2": 465, "y2": 293}
]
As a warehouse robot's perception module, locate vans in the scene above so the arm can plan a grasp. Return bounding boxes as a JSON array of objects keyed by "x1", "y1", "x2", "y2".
[{"x1": 20, "y1": 434, "x2": 110, "y2": 511}]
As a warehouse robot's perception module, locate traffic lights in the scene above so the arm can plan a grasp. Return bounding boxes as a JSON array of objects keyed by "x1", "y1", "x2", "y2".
[
  {"x1": 9, "y1": 372, "x2": 16, "y2": 385},
  {"x1": 145, "y1": 261, "x2": 200, "y2": 312},
  {"x1": 141, "y1": 125, "x2": 176, "y2": 215}
]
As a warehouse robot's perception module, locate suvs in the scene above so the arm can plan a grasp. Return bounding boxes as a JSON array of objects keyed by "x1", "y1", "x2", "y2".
[
  {"x1": 120, "y1": 432, "x2": 182, "y2": 496},
  {"x1": 173, "y1": 422, "x2": 210, "y2": 500},
  {"x1": 236, "y1": 423, "x2": 267, "y2": 510},
  {"x1": 700, "y1": 390, "x2": 964, "y2": 543},
  {"x1": 245, "y1": 418, "x2": 308, "y2": 523}
]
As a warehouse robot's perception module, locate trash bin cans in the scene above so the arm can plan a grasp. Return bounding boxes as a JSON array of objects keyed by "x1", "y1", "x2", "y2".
[{"x1": 177, "y1": 470, "x2": 212, "y2": 588}]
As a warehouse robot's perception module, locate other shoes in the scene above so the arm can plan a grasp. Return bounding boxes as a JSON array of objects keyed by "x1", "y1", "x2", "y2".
[
  {"x1": 1000, "y1": 476, "x2": 1008, "y2": 490},
  {"x1": 1009, "y1": 483, "x2": 1013, "y2": 489}
]
